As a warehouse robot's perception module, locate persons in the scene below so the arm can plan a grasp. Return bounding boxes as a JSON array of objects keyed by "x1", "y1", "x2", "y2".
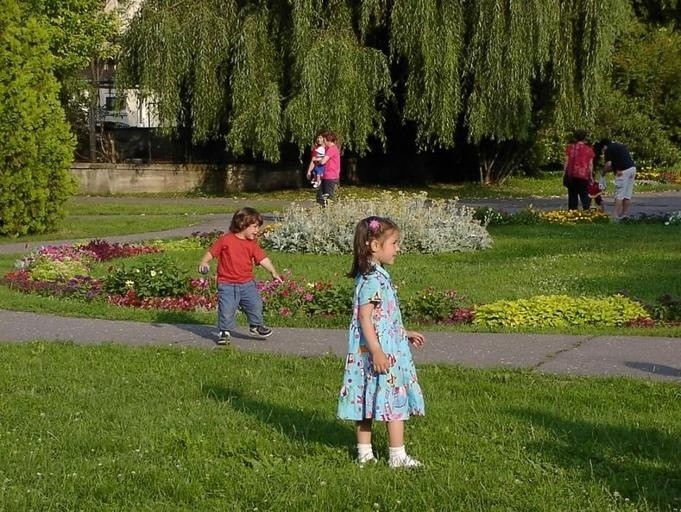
[
  {"x1": 599, "y1": 137, "x2": 636, "y2": 221},
  {"x1": 561, "y1": 127, "x2": 596, "y2": 211},
  {"x1": 581, "y1": 171, "x2": 606, "y2": 210},
  {"x1": 197, "y1": 206, "x2": 285, "y2": 344},
  {"x1": 335, "y1": 214, "x2": 428, "y2": 470},
  {"x1": 310, "y1": 146, "x2": 327, "y2": 189},
  {"x1": 306, "y1": 126, "x2": 341, "y2": 207},
  {"x1": 307, "y1": 130, "x2": 326, "y2": 203}
]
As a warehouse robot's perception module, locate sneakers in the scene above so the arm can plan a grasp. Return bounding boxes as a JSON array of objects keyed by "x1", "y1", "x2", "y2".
[
  {"x1": 249, "y1": 324, "x2": 273, "y2": 337},
  {"x1": 217, "y1": 330, "x2": 231, "y2": 344}
]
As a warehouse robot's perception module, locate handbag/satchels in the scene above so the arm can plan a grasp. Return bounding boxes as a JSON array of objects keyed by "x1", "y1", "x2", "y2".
[{"x1": 563, "y1": 176, "x2": 571, "y2": 187}]
